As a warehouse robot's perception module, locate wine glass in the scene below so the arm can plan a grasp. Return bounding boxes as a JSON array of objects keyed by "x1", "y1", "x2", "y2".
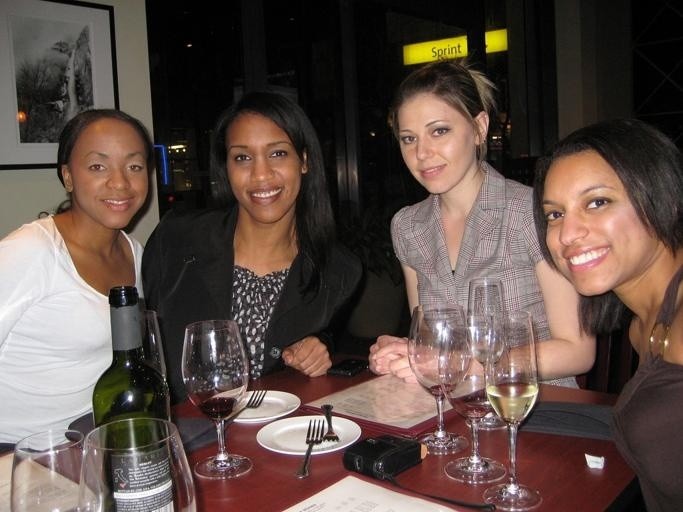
[
  {"x1": 139, "y1": 308, "x2": 167, "y2": 380},
  {"x1": 77, "y1": 417, "x2": 198, "y2": 512},
  {"x1": 483, "y1": 310, "x2": 543, "y2": 512},
  {"x1": 10, "y1": 428, "x2": 102, "y2": 512},
  {"x1": 466, "y1": 277, "x2": 509, "y2": 431},
  {"x1": 181, "y1": 319, "x2": 254, "y2": 480},
  {"x1": 437, "y1": 315, "x2": 508, "y2": 485},
  {"x1": 407, "y1": 302, "x2": 472, "y2": 456}
]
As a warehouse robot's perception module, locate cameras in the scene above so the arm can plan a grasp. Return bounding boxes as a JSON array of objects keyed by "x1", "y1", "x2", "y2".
[{"x1": 342, "y1": 435, "x2": 428, "y2": 482}]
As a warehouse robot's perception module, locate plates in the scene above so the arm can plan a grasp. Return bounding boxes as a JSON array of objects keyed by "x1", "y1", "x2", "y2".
[
  {"x1": 224, "y1": 390, "x2": 301, "y2": 424},
  {"x1": 255, "y1": 415, "x2": 362, "y2": 456}
]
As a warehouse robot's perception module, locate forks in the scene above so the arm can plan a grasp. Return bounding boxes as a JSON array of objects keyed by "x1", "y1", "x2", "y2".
[
  {"x1": 223, "y1": 387, "x2": 268, "y2": 430},
  {"x1": 295, "y1": 419, "x2": 324, "y2": 479},
  {"x1": 320, "y1": 404, "x2": 340, "y2": 442}
]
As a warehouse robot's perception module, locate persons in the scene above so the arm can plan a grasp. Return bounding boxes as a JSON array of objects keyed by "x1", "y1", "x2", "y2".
[
  {"x1": 141, "y1": 88, "x2": 363, "y2": 406},
  {"x1": 367, "y1": 54, "x2": 598, "y2": 390},
  {"x1": 532, "y1": 117, "x2": 683, "y2": 512},
  {"x1": 0, "y1": 108, "x2": 156, "y2": 457}
]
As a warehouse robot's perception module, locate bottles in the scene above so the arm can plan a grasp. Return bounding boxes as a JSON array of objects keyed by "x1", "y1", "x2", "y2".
[{"x1": 91, "y1": 285, "x2": 183, "y2": 512}]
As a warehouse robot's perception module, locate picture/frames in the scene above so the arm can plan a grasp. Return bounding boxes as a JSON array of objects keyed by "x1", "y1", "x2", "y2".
[{"x1": 0, "y1": 0, "x2": 119, "y2": 170}]
